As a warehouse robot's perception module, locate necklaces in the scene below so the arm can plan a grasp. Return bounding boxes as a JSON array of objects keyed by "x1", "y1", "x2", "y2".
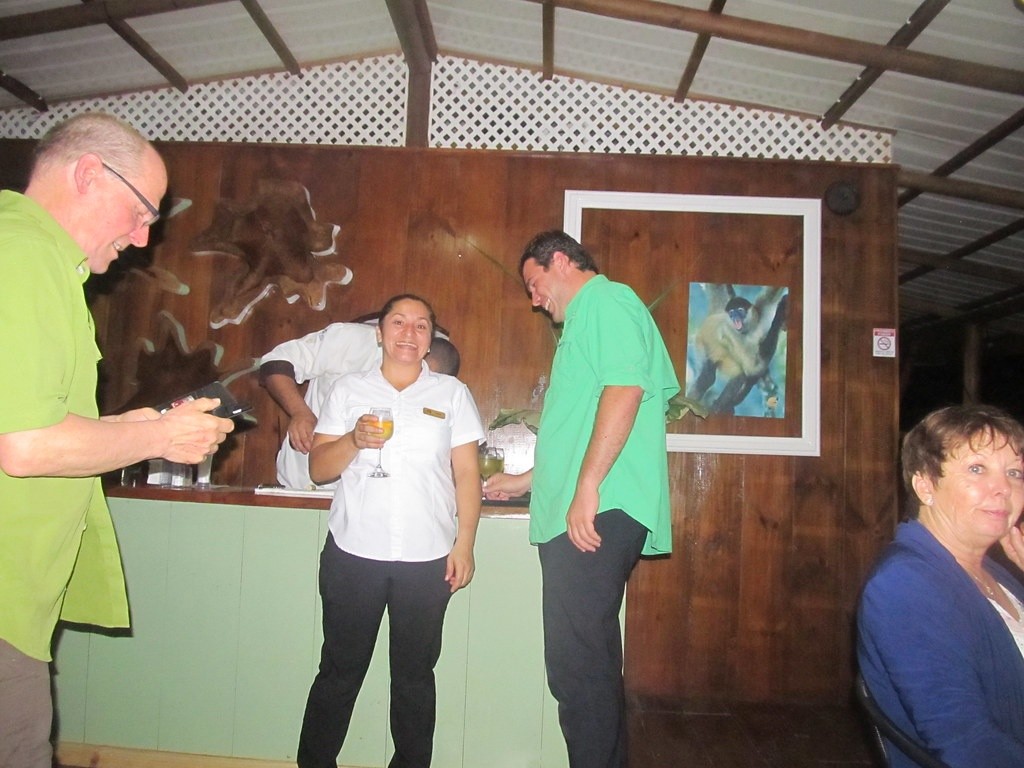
[{"x1": 956, "y1": 556, "x2": 997, "y2": 599}]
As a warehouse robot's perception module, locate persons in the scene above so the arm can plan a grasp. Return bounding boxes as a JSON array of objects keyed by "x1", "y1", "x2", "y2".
[
  {"x1": 260, "y1": 294, "x2": 487, "y2": 768},
  {"x1": 855, "y1": 403, "x2": 1024, "y2": 768},
  {"x1": 482, "y1": 230, "x2": 681, "y2": 768},
  {"x1": 0, "y1": 113, "x2": 235, "y2": 768}
]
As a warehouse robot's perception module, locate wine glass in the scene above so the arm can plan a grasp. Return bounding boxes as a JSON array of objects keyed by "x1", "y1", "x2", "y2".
[
  {"x1": 368, "y1": 408, "x2": 394, "y2": 477},
  {"x1": 479, "y1": 447, "x2": 497, "y2": 500}
]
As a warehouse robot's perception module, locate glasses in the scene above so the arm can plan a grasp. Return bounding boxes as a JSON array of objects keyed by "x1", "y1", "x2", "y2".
[{"x1": 101, "y1": 163, "x2": 160, "y2": 227}]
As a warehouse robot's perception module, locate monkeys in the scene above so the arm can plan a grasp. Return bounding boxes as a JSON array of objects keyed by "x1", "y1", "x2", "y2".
[{"x1": 686, "y1": 285, "x2": 789, "y2": 411}]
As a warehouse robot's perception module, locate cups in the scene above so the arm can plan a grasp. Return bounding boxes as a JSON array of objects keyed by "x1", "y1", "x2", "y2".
[{"x1": 496, "y1": 448, "x2": 504, "y2": 473}]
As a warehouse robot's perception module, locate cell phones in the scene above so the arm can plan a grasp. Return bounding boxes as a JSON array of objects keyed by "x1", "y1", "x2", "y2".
[{"x1": 154, "y1": 381, "x2": 253, "y2": 422}]
